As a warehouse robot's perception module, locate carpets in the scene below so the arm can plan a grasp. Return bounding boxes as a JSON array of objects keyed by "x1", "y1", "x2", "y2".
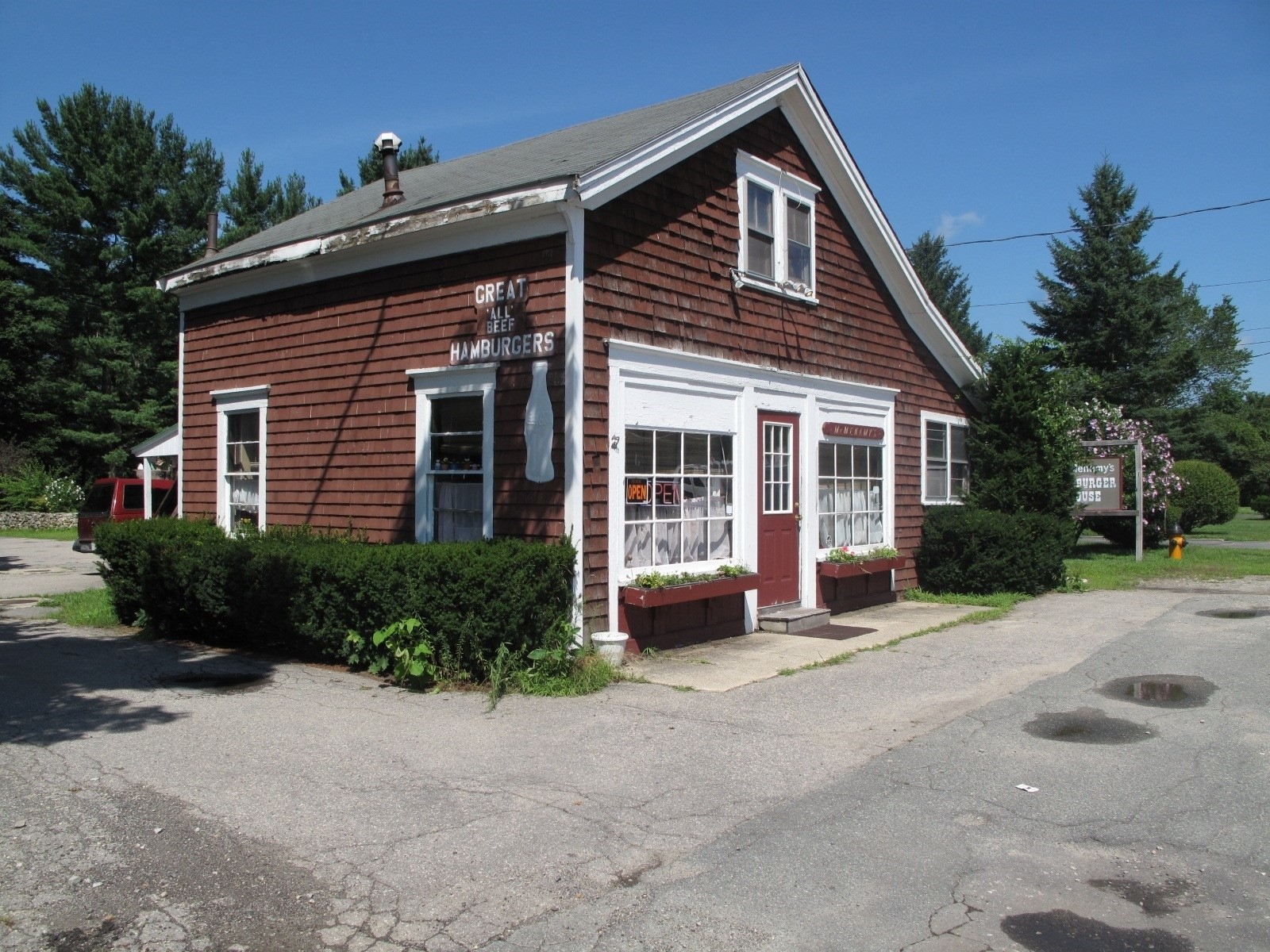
[{"x1": 788, "y1": 624, "x2": 879, "y2": 641}]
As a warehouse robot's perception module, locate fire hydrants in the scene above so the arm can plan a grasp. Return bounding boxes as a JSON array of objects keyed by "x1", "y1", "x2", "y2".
[{"x1": 1169, "y1": 520, "x2": 1189, "y2": 560}]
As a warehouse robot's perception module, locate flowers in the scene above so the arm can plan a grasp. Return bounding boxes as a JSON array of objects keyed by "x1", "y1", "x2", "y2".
[{"x1": 824, "y1": 542, "x2": 903, "y2": 565}]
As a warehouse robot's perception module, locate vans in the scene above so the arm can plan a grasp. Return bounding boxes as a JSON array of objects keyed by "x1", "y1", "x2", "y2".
[{"x1": 72, "y1": 476, "x2": 175, "y2": 554}]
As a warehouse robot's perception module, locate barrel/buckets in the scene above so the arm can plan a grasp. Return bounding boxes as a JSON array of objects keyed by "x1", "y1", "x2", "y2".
[{"x1": 591, "y1": 631, "x2": 629, "y2": 668}]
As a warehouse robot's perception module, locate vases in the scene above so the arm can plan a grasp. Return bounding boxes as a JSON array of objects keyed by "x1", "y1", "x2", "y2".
[{"x1": 820, "y1": 554, "x2": 907, "y2": 578}]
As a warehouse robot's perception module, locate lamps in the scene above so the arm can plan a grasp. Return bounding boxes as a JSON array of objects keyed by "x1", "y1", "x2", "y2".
[
  {"x1": 730, "y1": 269, "x2": 744, "y2": 289},
  {"x1": 780, "y1": 279, "x2": 803, "y2": 293},
  {"x1": 800, "y1": 282, "x2": 813, "y2": 297}
]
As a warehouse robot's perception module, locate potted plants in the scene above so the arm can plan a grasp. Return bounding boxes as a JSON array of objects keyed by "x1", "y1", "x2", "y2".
[{"x1": 623, "y1": 554, "x2": 763, "y2": 608}]
